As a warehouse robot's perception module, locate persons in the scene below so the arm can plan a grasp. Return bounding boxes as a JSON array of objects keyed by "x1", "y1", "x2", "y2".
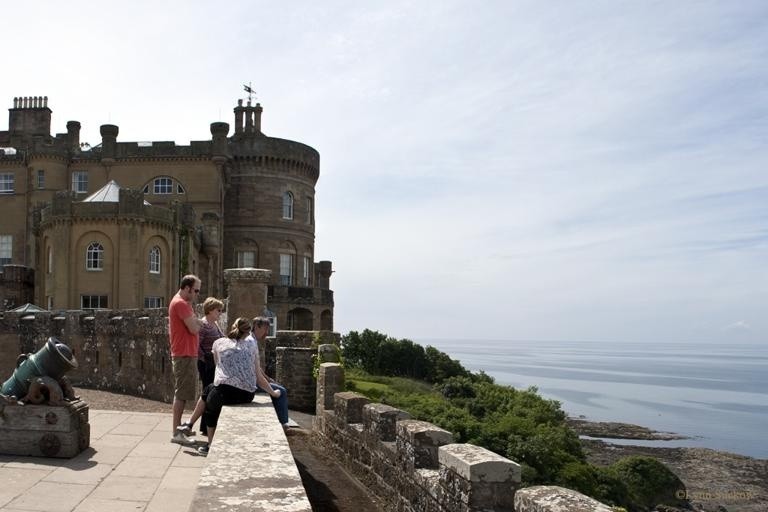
[
  {"x1": 178, "y1": 316, "x2": 281, "y2": 456},
  {"x1": 195, "y1": 296, "x2": 224, "y2": 387},
  {"x1": 167, "y1": 274, "x2": 204, "y2": 446},
  {"x1": 245, "y1": 316, "x2": 298, "y2": 440}
]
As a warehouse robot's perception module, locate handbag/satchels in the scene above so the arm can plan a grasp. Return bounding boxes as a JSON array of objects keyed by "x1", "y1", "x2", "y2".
[{"x1": 205, "y1": 351, "x2": 215, "y2": 373}]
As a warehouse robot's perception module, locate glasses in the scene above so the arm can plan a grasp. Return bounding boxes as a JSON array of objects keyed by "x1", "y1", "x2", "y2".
[{"x1": 194, "y1": 289, "x2": 200, "y2": 293}]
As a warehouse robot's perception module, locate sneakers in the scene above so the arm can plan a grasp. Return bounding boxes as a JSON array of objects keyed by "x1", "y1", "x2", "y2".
[
  {"x1": 197, "y1": 446, "x2": 209, "y2": 456},
  {"x1": 172, "y1": 423, "x2": 197, "y2": 445}
]
